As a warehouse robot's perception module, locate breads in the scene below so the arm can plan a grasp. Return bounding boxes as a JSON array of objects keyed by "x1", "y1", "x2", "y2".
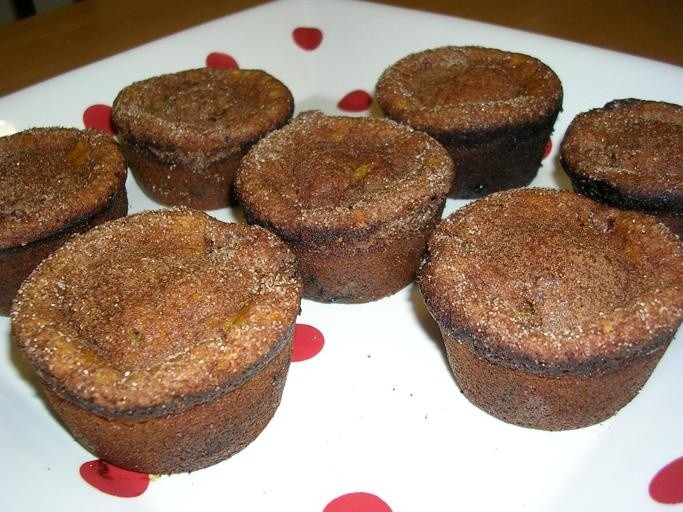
[
  {"x1": 11, "y1": 207, "x2": 305, "y2": 474},
  {"x1": 374, "y1": 45, "x2": 564, "y2": 200},
  {"x1": 418, "y1": 187, "x2": 683, "y2": 431},
  {"x1": 0, "y1": 125, "x2": 130, "y2": 318},
  {"x1": 235, "y1": 112, "x2": 456, "y2": 304},
  {"x1": 559, "y1": 99, "x2": 683, "y2": 242},
  {"x1": 110, "y1": 65, "x2": 294, "y2": 211}
]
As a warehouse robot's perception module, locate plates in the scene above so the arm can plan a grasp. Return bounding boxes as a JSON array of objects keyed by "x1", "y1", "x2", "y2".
[{"x1": 2, "y1": 0, "x2": 682, "y2": 509}]
